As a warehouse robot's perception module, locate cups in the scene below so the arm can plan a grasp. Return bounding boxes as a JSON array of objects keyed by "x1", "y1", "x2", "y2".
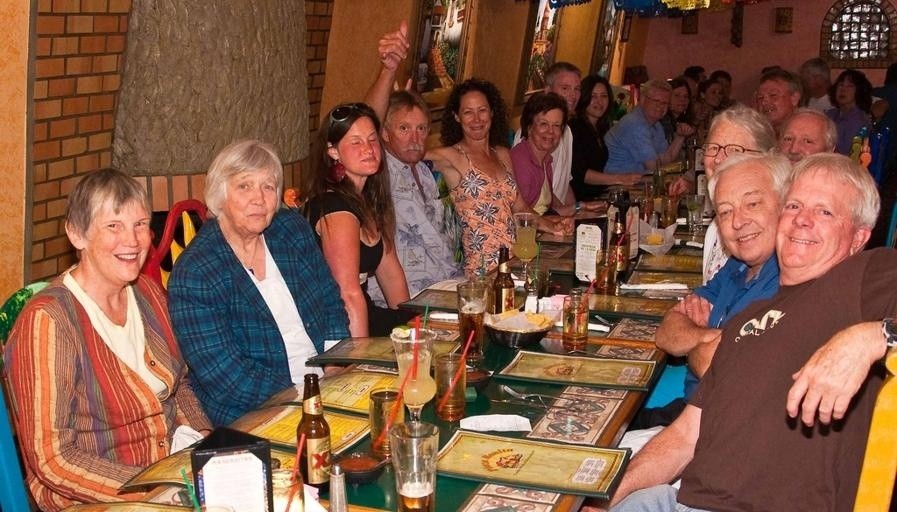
[
  {"x1": 270, "y1": 467, "x2": 305, "y2": 512},
  {"x1": 387, "y1": 421, "x2": 438, "y2": 512},
  {"x1": 455, "y1": 140, "x2": 705, "y2": 360},
  {"x1": 433, "y1": 352, "x2": 466, "y2": 421},
  {"x1": 172, "y1": 489, "x2": 194, "y2": 506},
  {"x1": 369, "y1": 387, "x2": 405, "y2": 456}
]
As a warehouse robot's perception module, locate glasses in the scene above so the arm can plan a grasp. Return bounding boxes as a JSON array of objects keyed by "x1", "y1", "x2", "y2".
[
  {"x1": 701, "y1": 142, "x2": 761, "y2": 158},
  {"x1": 329, "y1": 103, "x2": 368, "y2": 132},
  {"x1": 647, "y1": 98, "x2": 669, "y2": 106}
]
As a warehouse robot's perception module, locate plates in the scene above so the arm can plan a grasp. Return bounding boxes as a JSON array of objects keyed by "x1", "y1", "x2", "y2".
[
  {"x1": 331, "y1": 451, "x2": 384, "y2": 477},
  {"x1": 464, "y1": 370, "x2": 493, "y2": 392}
]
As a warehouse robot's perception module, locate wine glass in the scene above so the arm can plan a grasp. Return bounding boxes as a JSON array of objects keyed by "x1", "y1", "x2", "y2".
[{"x1": 391, "y1": 329, "x2": 438, "y2": 438}]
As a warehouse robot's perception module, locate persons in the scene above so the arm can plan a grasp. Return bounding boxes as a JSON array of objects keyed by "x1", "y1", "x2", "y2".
[
  {"x1": 423, "y1": 78, "x2": 575, "y2": 277},
  {"x1": 596, "y1": 150, "x2": 895, "y2": 511},
  {"x1": 606, "y1": 155, "x2": 795, "y2": 429},
  {"x1": 1, "y1": 167, "x2": 221, "y2": 512},
  {"x1": 504, "y1": 46, "x2": 897, "y2": 214},
  {"x1": 292, "y1": 99, "x2": 419, "y2": 343},
  {"x1": 167, "y1": 135, "x2": 351, "y2": 428},
  {"x1": 358, "y1": 20, "x2": 461, "y2": 311}
]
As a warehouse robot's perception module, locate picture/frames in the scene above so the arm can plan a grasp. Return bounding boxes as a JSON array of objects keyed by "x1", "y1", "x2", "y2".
[
  {"x1": 514, "y1": 0, "x2": 566, "y2": 108},
  {"x1": 681, "y1": 11, "x2": 699, "y2": 34},
  {"x1": 402, "y1": 0, "x2": 476, "y2": 123},
  {"x1": 589, "y1": 0, "x2": 634, "y2": 82}
]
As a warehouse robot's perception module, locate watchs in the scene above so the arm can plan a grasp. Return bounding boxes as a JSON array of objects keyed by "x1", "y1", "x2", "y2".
[{"x1": 880, "y1": 313, "x2": 896, "y2": 347}]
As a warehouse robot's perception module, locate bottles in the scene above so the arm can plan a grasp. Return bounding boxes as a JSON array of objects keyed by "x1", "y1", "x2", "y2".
[
  {"x1": 329, "y1": 463, "x2": 349, "y2": 511},
  {"x1": 296, "y1": 373, "x2": 331, "y2": 495}
]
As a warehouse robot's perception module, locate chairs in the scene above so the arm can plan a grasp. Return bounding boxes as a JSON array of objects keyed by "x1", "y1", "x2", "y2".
[
  {"x1": 0, "y1": 281, "x2": 77, "y2": 512},
  {"x1": 852, "y1": 345, "x2": 897, "y2": 512}
]
